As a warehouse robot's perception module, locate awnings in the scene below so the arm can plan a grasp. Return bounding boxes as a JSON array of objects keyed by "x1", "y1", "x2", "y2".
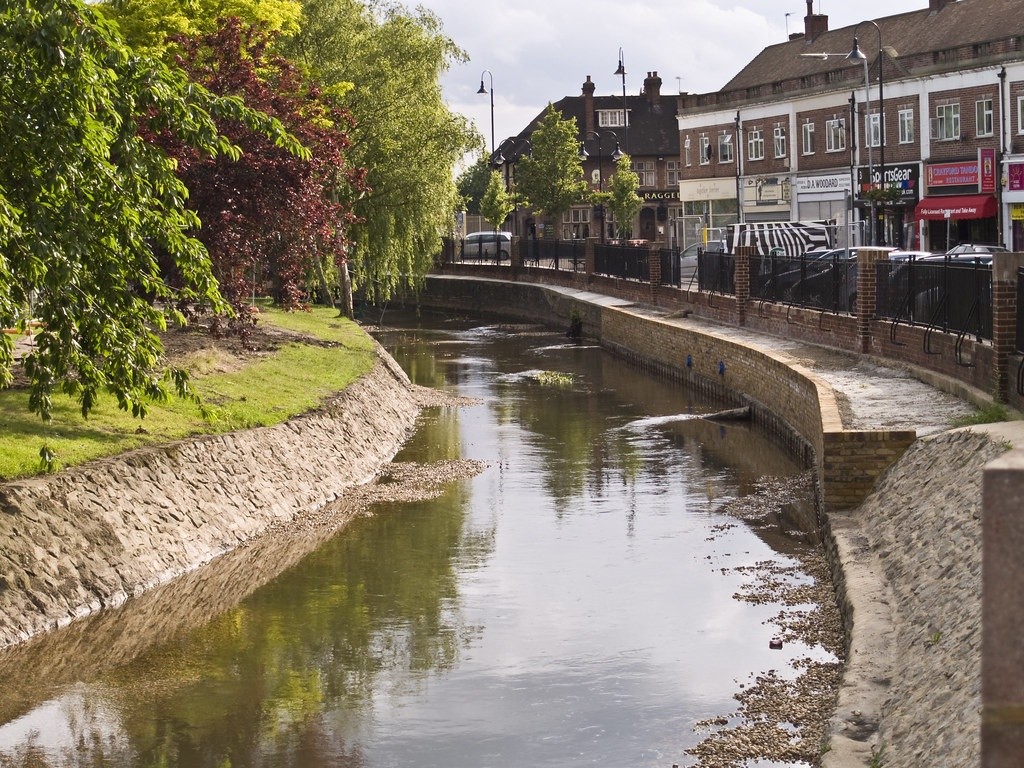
[{"x1": 915, "y1": 196, "x2": 999, "y2": 220}]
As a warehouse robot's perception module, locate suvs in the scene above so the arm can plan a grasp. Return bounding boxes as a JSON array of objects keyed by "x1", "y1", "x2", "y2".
[{"x1": 761, "y1": 242, "x2": 1011, "y2": 306}]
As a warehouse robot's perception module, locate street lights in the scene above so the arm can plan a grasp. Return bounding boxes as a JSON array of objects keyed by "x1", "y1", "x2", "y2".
[
  {"x1": 477, "y1": 69, "x2": 501, "y2": 265},
  {"x1": 843, "y1": 19, "x2": 887, "y2": 236},
  {"x1": 493, "y1": 137, "x2": 534, "y2": 237},
  {"x1": 613, "y1": 46, "x2": 629, "y2": 159},
  {"x1": 574, "y1": 129, "x2": 626, "y2": 242}
]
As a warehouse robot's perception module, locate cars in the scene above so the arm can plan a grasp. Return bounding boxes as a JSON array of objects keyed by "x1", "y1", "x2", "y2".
[{"x1": 674, "y1": 239, "x2": 728, "y2": 281}]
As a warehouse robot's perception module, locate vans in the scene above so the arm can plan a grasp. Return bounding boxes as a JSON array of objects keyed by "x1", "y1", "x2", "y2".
[{"x1": 462, "y1": 230, "x2": 513, "y2": 262}]
